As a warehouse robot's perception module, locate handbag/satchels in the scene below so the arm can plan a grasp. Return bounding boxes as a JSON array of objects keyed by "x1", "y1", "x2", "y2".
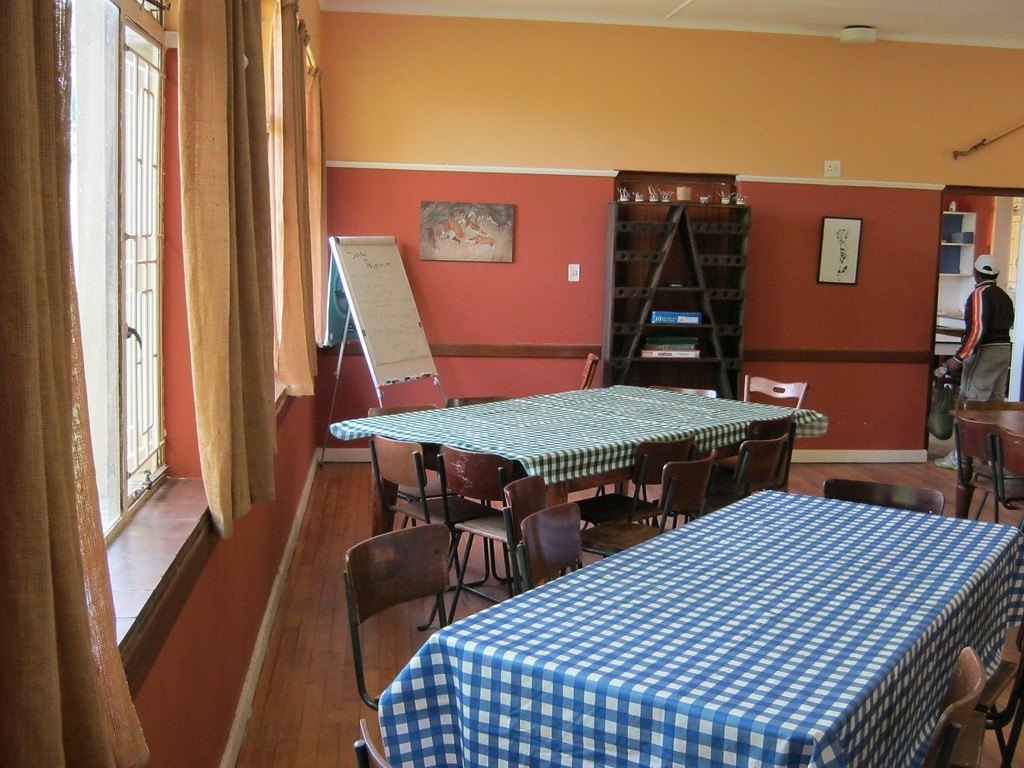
[{"x1": 928, "y1": 370, "x2": 954, "y2": 440}]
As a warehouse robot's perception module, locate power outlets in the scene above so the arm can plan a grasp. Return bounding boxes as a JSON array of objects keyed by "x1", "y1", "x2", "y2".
[{"x1": 823, "y1": 160, "x2": 842, "y2": 178}]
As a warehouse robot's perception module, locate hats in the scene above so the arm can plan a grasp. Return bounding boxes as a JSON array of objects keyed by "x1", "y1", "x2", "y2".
[{"x1": 974, "y1": 254, "x2": 999, "y2": 275}]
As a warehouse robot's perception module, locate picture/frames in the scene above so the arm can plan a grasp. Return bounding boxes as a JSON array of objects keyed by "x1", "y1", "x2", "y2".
[{"x1": 817, "y1": 216, "x2": 862, "y2": 286}]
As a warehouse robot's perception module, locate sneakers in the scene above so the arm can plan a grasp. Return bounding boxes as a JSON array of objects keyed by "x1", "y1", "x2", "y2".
[{"x1": 935, "y1": 451, "x2": 965, "y2": 471}]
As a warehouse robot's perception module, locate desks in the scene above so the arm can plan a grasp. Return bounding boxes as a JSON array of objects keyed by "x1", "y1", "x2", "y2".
[
  {"x1": 376, "y1": 489, "x2": 1024, "y2": 768},
  {"x1": 330, "y1": 384, "x2": 828, "y2": 584},
  {"x1": 951, "y1": 407, "x2": 1024, "y2": 521}
]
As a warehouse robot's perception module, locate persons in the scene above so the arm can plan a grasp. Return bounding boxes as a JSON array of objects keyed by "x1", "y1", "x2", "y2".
[{"x1": 935, "y1": 255, "x2": 1014, "y2": 470}]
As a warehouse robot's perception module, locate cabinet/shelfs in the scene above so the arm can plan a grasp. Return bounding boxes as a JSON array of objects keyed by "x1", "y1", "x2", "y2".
[
  {"x1": 601, "y1": 169, "x2": 751, "y2": 401},
  {"x1": 935, "y1": 212, "x2": 978, "y2": 355}
]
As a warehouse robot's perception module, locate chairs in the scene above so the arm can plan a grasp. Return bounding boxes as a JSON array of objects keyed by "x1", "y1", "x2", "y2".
[{"x1": 330, "y1": 352, "x2": 1024, "y2": 768}]
